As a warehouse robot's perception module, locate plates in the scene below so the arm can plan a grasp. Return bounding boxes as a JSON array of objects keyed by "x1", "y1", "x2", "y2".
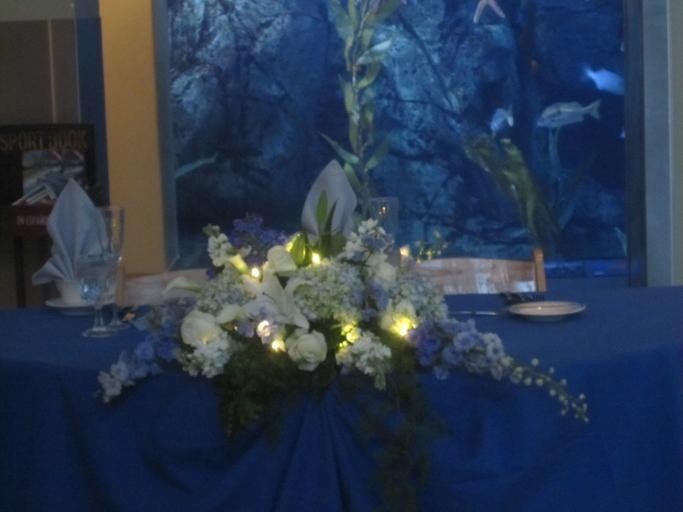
[
  {"x1": 43, "y1": 296, "x2": 117, "y2": 317},
  {"x1": 507, "y1": 301, "x2": 589, "y2": 322}
]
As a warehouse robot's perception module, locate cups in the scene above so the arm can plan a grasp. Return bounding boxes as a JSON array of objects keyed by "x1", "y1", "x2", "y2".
[
  {"x1": 367, "y1": 195, "x2": 403, "y2": 241},
  {"x1": 54, "y1": 278, "x2": 82, "y2": 304}
]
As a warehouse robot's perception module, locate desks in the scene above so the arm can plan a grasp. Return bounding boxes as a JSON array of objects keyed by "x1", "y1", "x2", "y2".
[{"x1": 0, "y1": 284, "x2": 681, "y2": 511}]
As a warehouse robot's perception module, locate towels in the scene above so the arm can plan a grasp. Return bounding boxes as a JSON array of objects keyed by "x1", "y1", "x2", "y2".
[{"x1": 31, "y1": 178, "x2": 132, "y2": 290}]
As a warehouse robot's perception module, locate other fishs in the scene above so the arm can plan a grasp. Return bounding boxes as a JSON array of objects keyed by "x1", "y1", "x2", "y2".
[
  {"x1": 581, "y1": 62, "x2": 625, "y2": 96},
  {"x1": 535, "y1": 99, "x2": 601, "y2": 129},
  {"x1": 489, "y1": 104, "x2": 515, "y2": 133}
]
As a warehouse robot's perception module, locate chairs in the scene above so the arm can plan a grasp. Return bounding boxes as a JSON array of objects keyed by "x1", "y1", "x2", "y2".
[
  {"x1": 414, "y1": 246, "x2": 548, "y2": 296},
  {"x1": 115, "y1": 266, "x2": 211, "y2": 308}
]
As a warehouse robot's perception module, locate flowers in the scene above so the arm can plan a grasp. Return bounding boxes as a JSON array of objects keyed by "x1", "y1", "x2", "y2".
[{"x1": 91, "y1": 159, "x2": 591, "y2": 512}]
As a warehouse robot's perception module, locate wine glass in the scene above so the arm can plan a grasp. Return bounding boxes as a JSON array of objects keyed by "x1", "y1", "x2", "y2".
[{"x1": 69, "y1": 205, "x2": 128, "y2": 341}]
